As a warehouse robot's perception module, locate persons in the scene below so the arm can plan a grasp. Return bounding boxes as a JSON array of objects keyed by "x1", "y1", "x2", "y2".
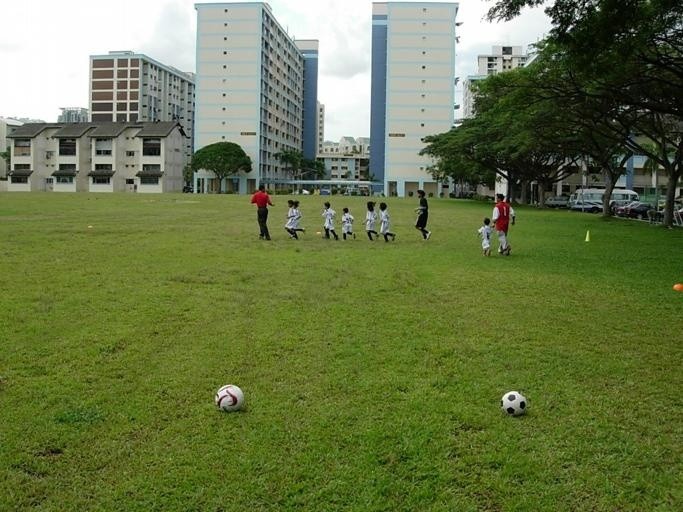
[
  {"x1": 378, "y1": 202, "x2": 396, "y2": 242},
  {"x1": 251, "y1": 184, "x2": 275, "y2": 241},
  {"x1": 320, "y1": 201, "x2": 338, "y2": 240},
  {"x1": 413, "y1": 189, "x2": 432, "y2": 240},
  {"x1": 361, "y1": 201, "x2": 380, "y2": 241},
  {"x1": 284, "y1": 199, "x2": 297, "y2": 239},
  {"x1": 338, "y1": 207, "x2": 356, "y2": 240},
  {"x1": 493, "y1": 193, "x2": 515, "y2": 256},
  {"x1": 476, "y1": 217, "x2": 495, "y2": 256},
  {"x1": 293, "y1": 200, "x2": 304, "y2": 239}
]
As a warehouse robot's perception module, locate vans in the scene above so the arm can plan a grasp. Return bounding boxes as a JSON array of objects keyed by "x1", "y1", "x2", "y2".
[{"x1": 544, "y1": 196, "x2": 568, "y2": 208}]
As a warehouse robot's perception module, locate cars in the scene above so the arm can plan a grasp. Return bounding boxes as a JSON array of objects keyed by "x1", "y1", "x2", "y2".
[
  {"x1": 567, "y1": 194, "x2": 575, "y2": 208},
  {"x1": 293, "y1": 185, "x2": 372, "y2": 197},
  {"x1": 448, "y1": 188, "x2": 476, "y2": 198},
  {"x1": 616, "y1": 199, "x2": 682, "y2": 222}
]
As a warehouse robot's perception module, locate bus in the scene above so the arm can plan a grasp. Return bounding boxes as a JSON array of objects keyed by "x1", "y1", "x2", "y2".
[{"x1": 571, "y1": 188, "x2": 640, "y2": 210}]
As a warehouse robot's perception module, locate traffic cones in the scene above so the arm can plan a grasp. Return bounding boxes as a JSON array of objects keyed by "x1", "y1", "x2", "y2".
[{"x1": 584, "y1": 230, "x2": 591, "y2": 242}]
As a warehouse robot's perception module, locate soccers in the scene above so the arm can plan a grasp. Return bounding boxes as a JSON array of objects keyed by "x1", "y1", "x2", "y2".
[
  {"x1": 215, "y1": 385, "x2": 244, "y2": 413},
  {"x1": 501, "y1": 391, "x2": 527, "y2": 415}
]
